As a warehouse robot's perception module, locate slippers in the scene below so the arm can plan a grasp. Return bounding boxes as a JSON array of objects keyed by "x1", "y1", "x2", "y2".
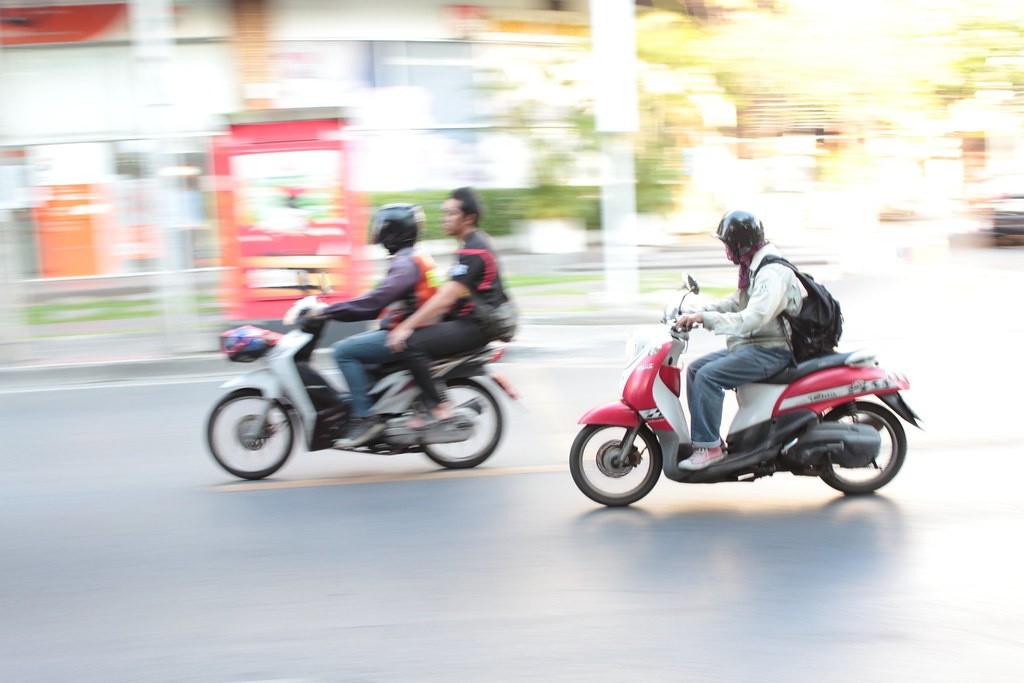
[{"x1": 408, "y1": 412, "x2": 460, "y2": 432}]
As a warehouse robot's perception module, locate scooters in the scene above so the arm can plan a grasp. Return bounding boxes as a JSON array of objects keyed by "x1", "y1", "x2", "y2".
[
  {"x1": 569, "y1": 274, "x2": 925, "y2": 507},
  {"x1": 205, "y1": 287, "x2": 519, "y2": 479}
]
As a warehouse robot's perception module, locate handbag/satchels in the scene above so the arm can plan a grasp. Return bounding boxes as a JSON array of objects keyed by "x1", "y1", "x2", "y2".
[{"x1": 480, "y1": 289, "x2": 519, "y2": 342}]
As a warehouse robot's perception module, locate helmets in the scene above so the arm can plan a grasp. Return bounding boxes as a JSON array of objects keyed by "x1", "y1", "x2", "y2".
[
  {"x1": 370, "y1": 203, "x2": 418, "y2": 243},
  {"x1": 710, "y1": 208, "x2": 765, "y2": 265}
]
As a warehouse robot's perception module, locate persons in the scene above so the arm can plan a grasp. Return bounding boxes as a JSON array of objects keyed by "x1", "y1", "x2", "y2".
[
  {"x1": 675, "y1": 208, "x2": 804, "y2": 472},
  {"x1": 384, "y1": 186, "x2": 511, "y2": 432},
  {"x1": 304, "y1": 204, "x2": 441, "y2": 451}
]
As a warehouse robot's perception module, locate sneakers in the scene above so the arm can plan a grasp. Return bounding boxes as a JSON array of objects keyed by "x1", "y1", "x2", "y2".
[
  {"x1": 337, "y1": 415, "x2": 387, "y2": 449},
  {"x1": 678, "y1": 436, "x2": 727, "y2": 471}
]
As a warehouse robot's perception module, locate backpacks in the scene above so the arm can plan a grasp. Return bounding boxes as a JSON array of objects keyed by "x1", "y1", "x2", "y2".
[{"x1": 750, "y1": 255, "x2": 844, "y2": 364}]
